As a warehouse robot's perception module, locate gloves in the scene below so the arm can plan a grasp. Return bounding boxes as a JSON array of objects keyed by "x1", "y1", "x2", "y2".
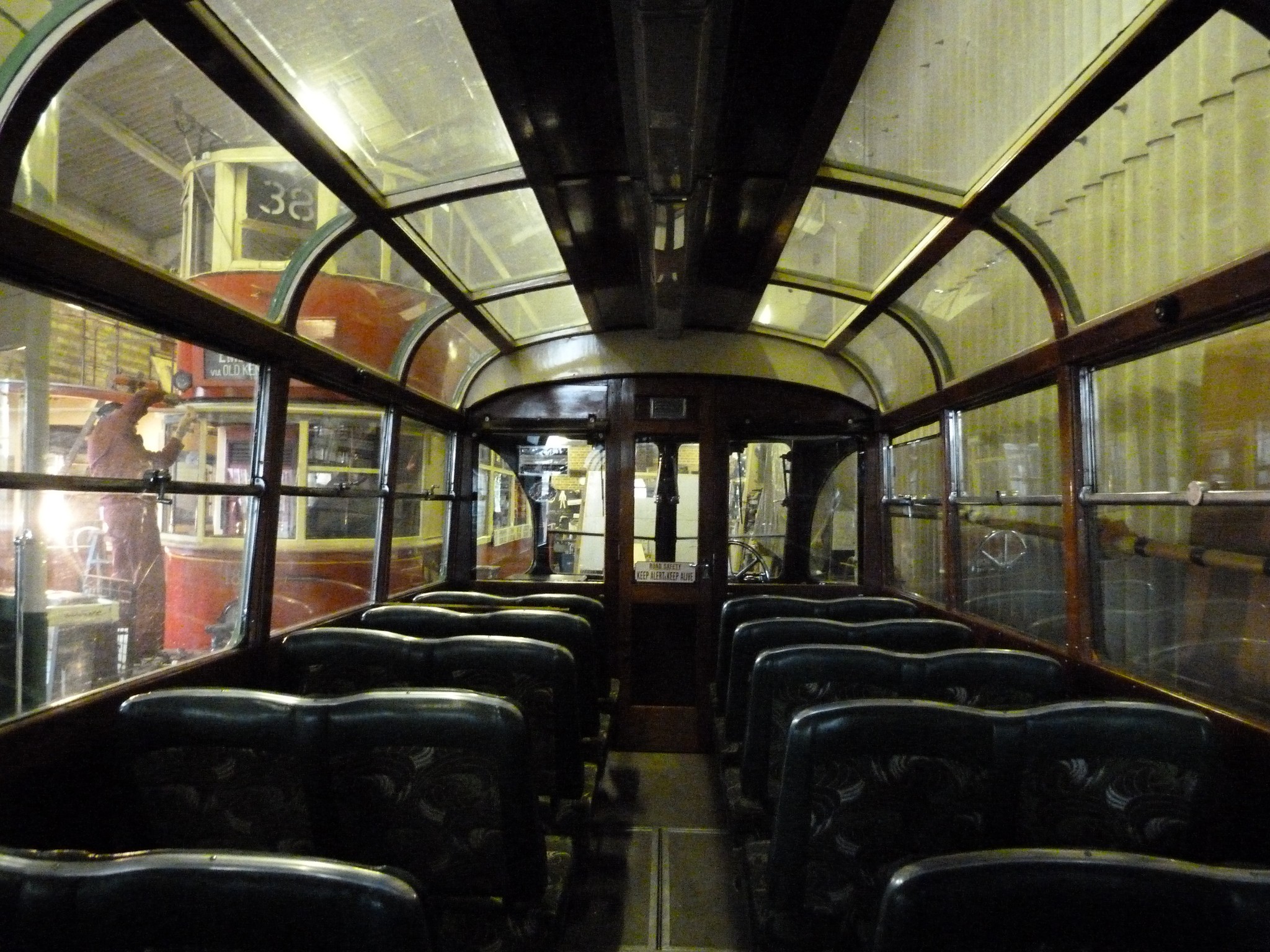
[{"x1": 173, "y1": 410, "x2": 199, "y2": 438}]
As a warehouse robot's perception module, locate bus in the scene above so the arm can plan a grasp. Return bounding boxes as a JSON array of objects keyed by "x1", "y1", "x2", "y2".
[
  {"x1": 2, "y1": 380, "x2": 176, "y2": 608},
  {"x1": 157, "y1": 142, "x2": 547, "y2": 660}
]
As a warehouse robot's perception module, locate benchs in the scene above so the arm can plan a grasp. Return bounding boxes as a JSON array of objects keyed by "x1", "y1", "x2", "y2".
[
  {"x1": 713, "y1": 594, "x2": 1270, "y2": 952},
  {"x1": 0, "y1": 587, "x2": 618, "y2": 952}
]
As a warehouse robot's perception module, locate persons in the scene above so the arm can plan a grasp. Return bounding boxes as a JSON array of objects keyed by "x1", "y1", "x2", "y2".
[{"x1": 87, "y1": 388, "x2": 198, "y2": 668}]
